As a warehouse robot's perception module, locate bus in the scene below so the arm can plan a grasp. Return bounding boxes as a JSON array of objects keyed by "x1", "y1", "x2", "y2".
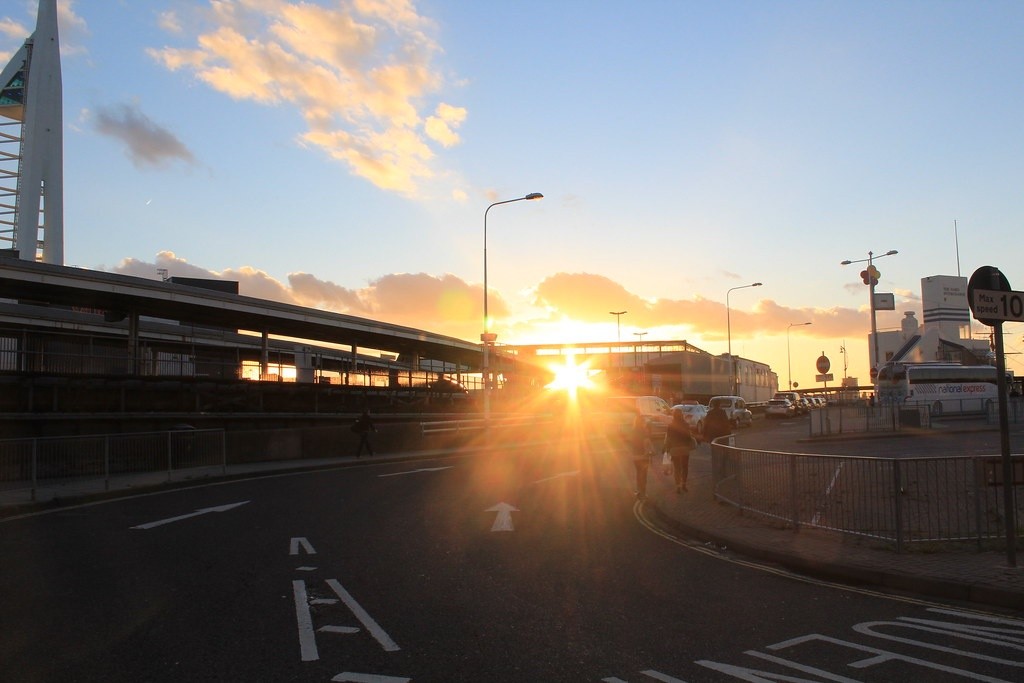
[{"x1": 877, "y1": 364, "x2": 1010, "y2": 416}]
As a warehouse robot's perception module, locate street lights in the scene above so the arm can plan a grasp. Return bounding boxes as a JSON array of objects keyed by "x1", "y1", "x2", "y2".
[
  {"x1": 841, "y1": 250, "x2": 898, "y2": 365},
  {"x1": 484, "y1": 193, "x2": 544, "y2": 415},
  {"x1": 840, "y1": 346, "x2": 846, "y2": 378},
  {"x1": 727, "y1": 283, "x2": 762, "y2": 396},
  {"x1": 610, "y1": 311, "x2": 627, "y2": 351},
  {"x1": 787, "y1": 322, "x2": 812, "y2": 392},
  {"x1": 634, "y1": 332, "x2": 647, "y2": 352}
]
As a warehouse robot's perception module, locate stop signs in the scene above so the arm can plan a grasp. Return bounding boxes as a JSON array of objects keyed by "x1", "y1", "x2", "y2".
[{"x1": 817, "y1": 356, "x2": 830, "y2": 374}]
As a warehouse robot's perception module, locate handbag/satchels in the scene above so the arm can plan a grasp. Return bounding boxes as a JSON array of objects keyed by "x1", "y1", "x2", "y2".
[
  {"x1": 644, "y1": 437, "x2": 657, "y2": 455},
  {"x1": 662, "y1": 451, "x2": 672, "y2": 475},
  {"x1": 351, "y1": 421, "x2": 361, "y2": 432},
  {"x1": 688, "y1": 436, "x2": 697, "y2": 451}
]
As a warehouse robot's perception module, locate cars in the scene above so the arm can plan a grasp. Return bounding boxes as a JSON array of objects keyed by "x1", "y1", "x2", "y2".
[
  {"x1": 766, "y1": 399, "x2": 795, "y2": 418},
  {"x1": 670, "y1": 404, "x2": 708, "y2": 434},
  {"x1": 709, "y1": 396, "x2": 752, "y2": 428},
  {"x1": 772, "y1": 392, "x2": 827, "y2": 414}
]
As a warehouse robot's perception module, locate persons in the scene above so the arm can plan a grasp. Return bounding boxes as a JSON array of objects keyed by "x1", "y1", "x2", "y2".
[
  {"x1": 661, "y1": 408, "x2": 692, "y2": 494},
  {"x1": 703, "y1": 399, "x2": 732, "y2": 444},
  {"x1": 356, "y1": 409, "x2": 378, "y2": 458},
  {"x1": 1010, "y1": 389, "x2": 1020, "y2": 410},
  {"x1": 631, "y1": 416, "x2": 654, "y2": 497}
]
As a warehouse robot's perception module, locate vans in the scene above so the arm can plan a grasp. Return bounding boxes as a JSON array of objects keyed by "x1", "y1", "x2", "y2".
[{"x1": 603, "y1": 396, "x2": 671, "y2": 443}]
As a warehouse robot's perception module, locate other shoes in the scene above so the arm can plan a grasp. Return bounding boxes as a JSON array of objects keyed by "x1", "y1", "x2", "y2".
[
  {"x1": 371, "y1": 451, "x2": 376, "y2": 456},
  {"x1": 635, "y1": 490, "x2": 640, "y2": 496},
  {"x1": 642, "y1": 495, "x2": 648, "y2": 498},
  {"x1": 676, "y1": 485, "x2": 681, "y2": 493},
  {"x1": 682, "y1": 484, "x2": 689, "y2": 492}
]
